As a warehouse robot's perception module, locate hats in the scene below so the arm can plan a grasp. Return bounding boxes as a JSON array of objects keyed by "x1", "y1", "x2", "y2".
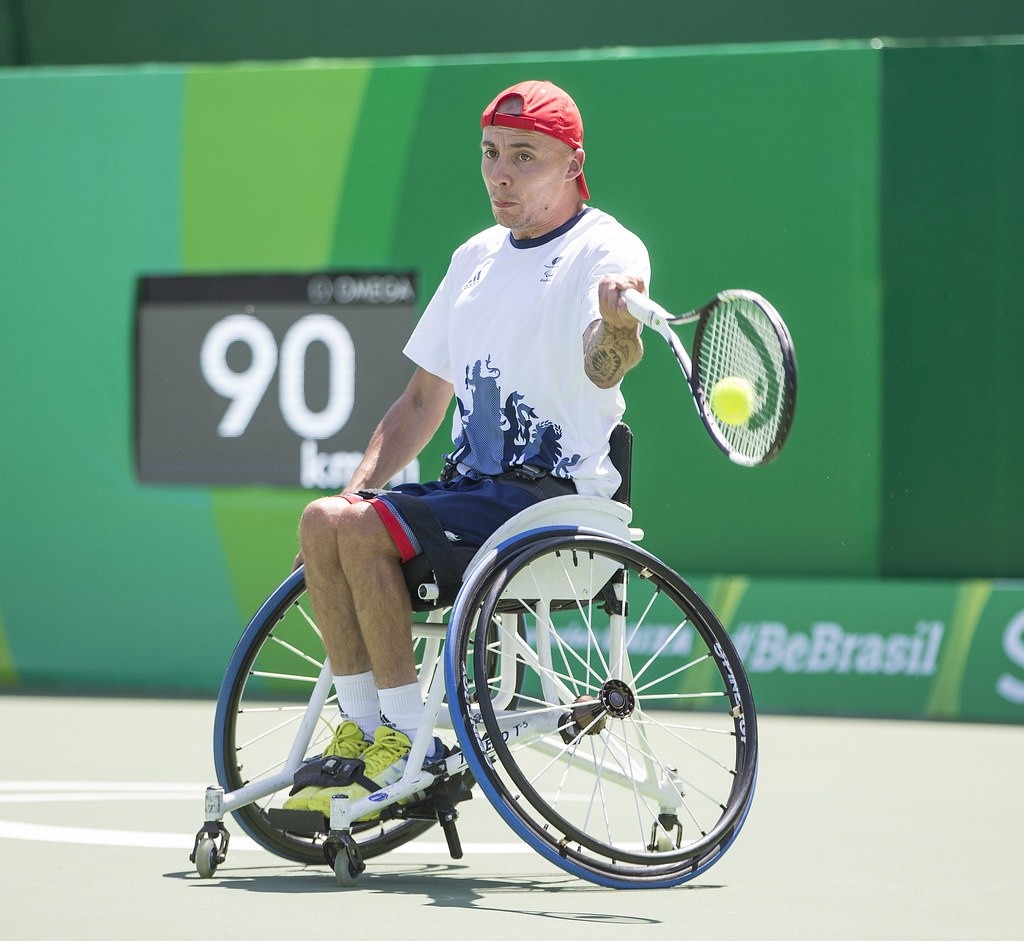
[{"x1": 482, "y1": 81, "x2": 590, "y2": 200}]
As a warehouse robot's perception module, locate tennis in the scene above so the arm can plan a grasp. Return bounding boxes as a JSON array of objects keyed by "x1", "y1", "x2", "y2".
[{"x1": 709, "y1": 375, "x2": 758, "y2": 428}]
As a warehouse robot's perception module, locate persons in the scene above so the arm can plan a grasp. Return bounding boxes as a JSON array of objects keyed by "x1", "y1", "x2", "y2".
[{"x1": 281, "y1": 79, "x2": 652, "y2": 824}]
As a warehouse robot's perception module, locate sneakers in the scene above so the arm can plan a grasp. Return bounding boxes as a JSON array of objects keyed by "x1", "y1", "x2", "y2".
[
  {"x1": 282, "y1": 715, "x2": 374, "y2": 811},
  {"x1": 308, "y1": 725, "x2": 450, "y2": 822}
]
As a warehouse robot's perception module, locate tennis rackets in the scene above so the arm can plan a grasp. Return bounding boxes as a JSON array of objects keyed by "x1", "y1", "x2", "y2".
[{"x1": 617, "y1": 282, "x2": 799, "y2": 469}]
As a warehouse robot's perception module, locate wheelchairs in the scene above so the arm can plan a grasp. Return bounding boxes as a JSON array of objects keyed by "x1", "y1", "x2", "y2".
[{"x1": 189, "y1": 422, "x2": 758, "y2": 889}]
